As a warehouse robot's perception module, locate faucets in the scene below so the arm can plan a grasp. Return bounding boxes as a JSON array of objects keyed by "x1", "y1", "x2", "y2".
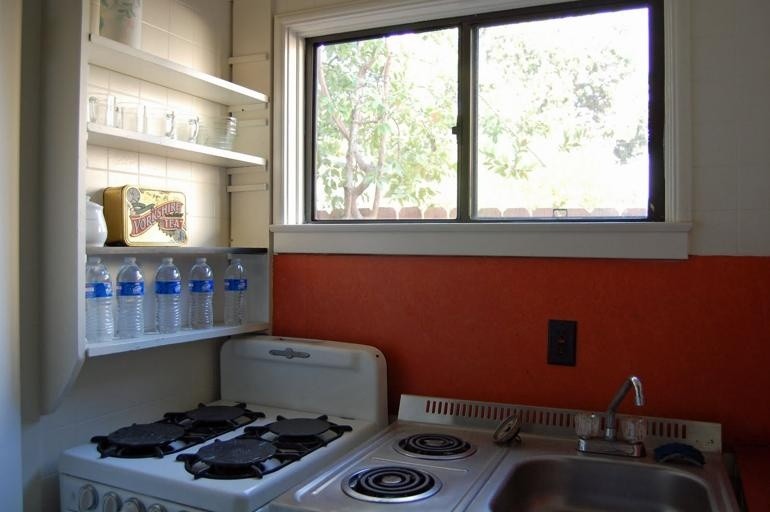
[{"x1": 606, "y1": 375, "x2": 644, "y2": 440}]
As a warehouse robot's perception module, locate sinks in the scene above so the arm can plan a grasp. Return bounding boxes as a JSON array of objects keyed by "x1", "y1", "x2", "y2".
[{"x1": 462, "y1": 439, "x2": 728, "y2": 511}]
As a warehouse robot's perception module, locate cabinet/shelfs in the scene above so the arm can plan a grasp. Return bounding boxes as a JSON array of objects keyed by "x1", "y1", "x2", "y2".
[{"x1": 41, "y1": 1, "x2": 273, "y2": 416}]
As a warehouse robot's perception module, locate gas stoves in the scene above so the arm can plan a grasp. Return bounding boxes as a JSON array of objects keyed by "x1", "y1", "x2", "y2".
[
  {"x1": 268, "y1": 417, "x2": 516, "y2": 511},
  {"x1": 57, "y1": 396, "x2": 367, "y2": 512}
]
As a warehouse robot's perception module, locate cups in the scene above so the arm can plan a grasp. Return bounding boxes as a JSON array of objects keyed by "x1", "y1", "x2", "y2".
[{"x1": 88, "y1": 95, "x2": 200, "y2": 144}]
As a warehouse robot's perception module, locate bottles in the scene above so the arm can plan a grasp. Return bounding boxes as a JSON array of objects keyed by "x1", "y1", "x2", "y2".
[
  {"x1": 154, "y1": 257, "x2": 183, "y2": 334},
  {"x1": 116, "y1": 257, "x2": 146, "y2": 340},
  {"x1": 222, "y1": 256, "x2": 250, "y2": 326},
  {"x1": 85, "y1": 258, "x2": 115, "y2": 343},
  {"x1": 188, "y1": 256, "x2": 215, "y2": 331}
]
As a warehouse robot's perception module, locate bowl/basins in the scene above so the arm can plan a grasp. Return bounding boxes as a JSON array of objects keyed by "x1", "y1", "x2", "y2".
[{"x1": 198, "y1": 113, "x2": 237, "y2": 148}]
